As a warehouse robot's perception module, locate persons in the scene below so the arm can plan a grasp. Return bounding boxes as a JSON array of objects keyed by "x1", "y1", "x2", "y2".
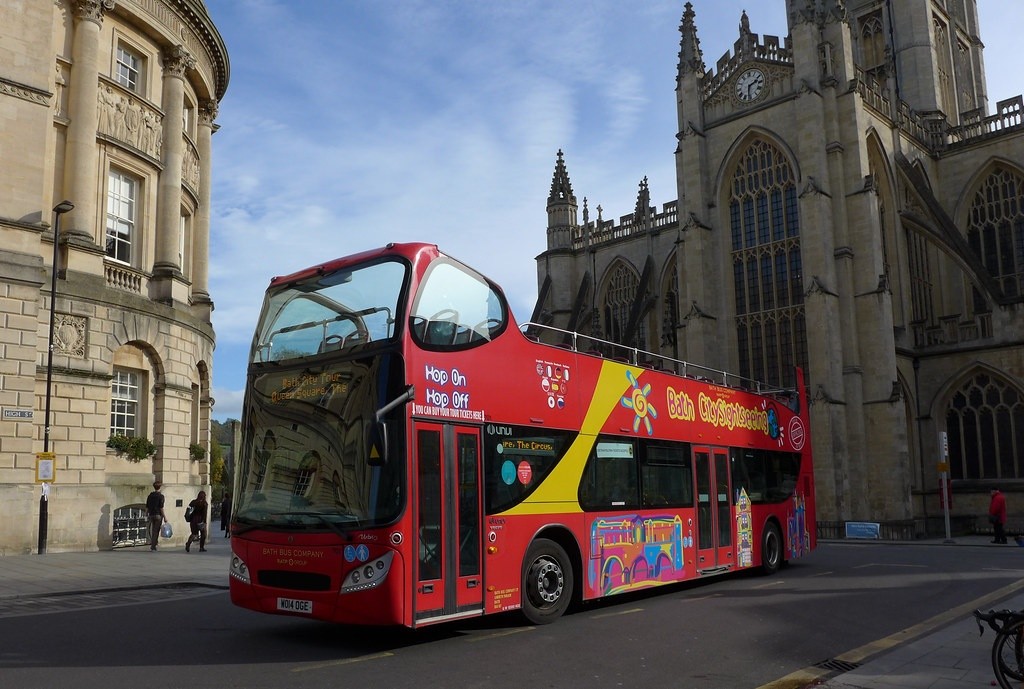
[
  {"x1": 220, "y1": 493, "x2": 232, "y2": 539},
  {"x1": 986, "y1": 484, "x2": 1008, "y2": 544},
  {"x1": 184, "y1": 491, "x2": 208, "y2": 553},
  {"x1": 142, "y1": 481, "x2": 168, "y2": 552}
]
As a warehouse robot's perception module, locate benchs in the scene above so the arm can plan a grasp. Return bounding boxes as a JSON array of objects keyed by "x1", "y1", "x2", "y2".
[{"x1": 970, "y1": 606, "x2": 1024, "y2": 689}]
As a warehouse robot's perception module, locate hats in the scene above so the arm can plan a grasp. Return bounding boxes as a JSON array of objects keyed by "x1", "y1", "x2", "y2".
[{"x1": 990, "y1": 485, "x2": 1001, "y2": 490}]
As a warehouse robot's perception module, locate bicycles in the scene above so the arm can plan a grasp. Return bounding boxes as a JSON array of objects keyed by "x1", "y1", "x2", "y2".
[{"x1": 971, "y1": 608, "x2": 1024, "y2": 689}]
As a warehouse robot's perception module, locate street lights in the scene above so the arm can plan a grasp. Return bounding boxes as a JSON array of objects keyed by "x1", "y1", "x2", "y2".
[{"x1": 37, "y1": 199, "x2": 75, "y2": 556}]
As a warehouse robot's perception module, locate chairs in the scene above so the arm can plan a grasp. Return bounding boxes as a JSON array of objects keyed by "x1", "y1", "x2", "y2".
[
  {"x1": 343, "y1": 331, "x2": 370, "y2": 350},
  {"x1": 679, "y1": 373, "x2": 697, "y2": 380},
  {"x1": 647, "y1": 494, "x2": 669, "y2": 505},
  {"x1": 317, "y1": 335, "x2": 343, "y2": 354},
  {"x1": 412, "y1": 316, "x2": 427, "y2": 343},
  {"x1": 555, "y1": 344, "x2": 574, "y2": 350},
  {"x1": 639, "y1": 362, "x2": 653, "y2": 370},
  {"x1": 612, "y1": 356, "x2": 630, "y2": 363},
  {"x1": 425, "y1": 309, "x2": 459, "y2": 346},
  {"x1": 717, "y1": 382, "x2": 732, "y2": 387},
  {"x1": 731, "y1": 386, "x2": 747, "y2": 392},
  {"x1": 526, "y1": 334, "x2": 540, "y2": 343},
  {"x1": 700, "y1": 377, "x2": 714, "y2": 384},
  {"x1": 472, "y1": 319, "x2": 503, "y2": 343},
  {"x1": 659, "y1": 367, "x2": 675, "y2": 374},
  {"x1": 455, "y1": 324, "x2": 473, "y2": 345},
  {"x1": 584, "y1": 351, "x2": 602, "y2": 357}
]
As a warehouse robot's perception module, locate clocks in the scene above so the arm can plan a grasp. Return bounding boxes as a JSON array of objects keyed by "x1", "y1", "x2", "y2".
[{"x1": 734, "y1": 69, "x2": 765, "y2": 103}]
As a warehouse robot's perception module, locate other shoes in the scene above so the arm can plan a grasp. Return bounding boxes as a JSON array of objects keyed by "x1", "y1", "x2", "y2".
[
  {"x1": 151, "y1": 546, "x2": 156, "y2": 551},
  {"x1": 186, "y1": 542, "x2": 190, "y2": 552},
  {"x1": 1000, "y1": 540, "x2": 1007, "y2": 544},
  {"x1": 991, "y1": 539, "x2": 1001, "y2": 543},
  {"x1": 199, "y1": 547, "x2": 207, "y2": 552}
]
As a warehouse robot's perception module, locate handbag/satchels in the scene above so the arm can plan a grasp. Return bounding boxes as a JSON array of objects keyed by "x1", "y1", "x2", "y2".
[
  {"x1": 184, "y1": 500, "x2": 196, "y2": 522},
  {"x1": 161, "y1": 523, "x2": 172, "y2": 537}
]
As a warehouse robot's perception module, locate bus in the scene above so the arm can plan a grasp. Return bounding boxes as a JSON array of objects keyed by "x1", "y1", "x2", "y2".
[{"x1": 230, "y1": 238, "x2": 817, "y2": 634}]
